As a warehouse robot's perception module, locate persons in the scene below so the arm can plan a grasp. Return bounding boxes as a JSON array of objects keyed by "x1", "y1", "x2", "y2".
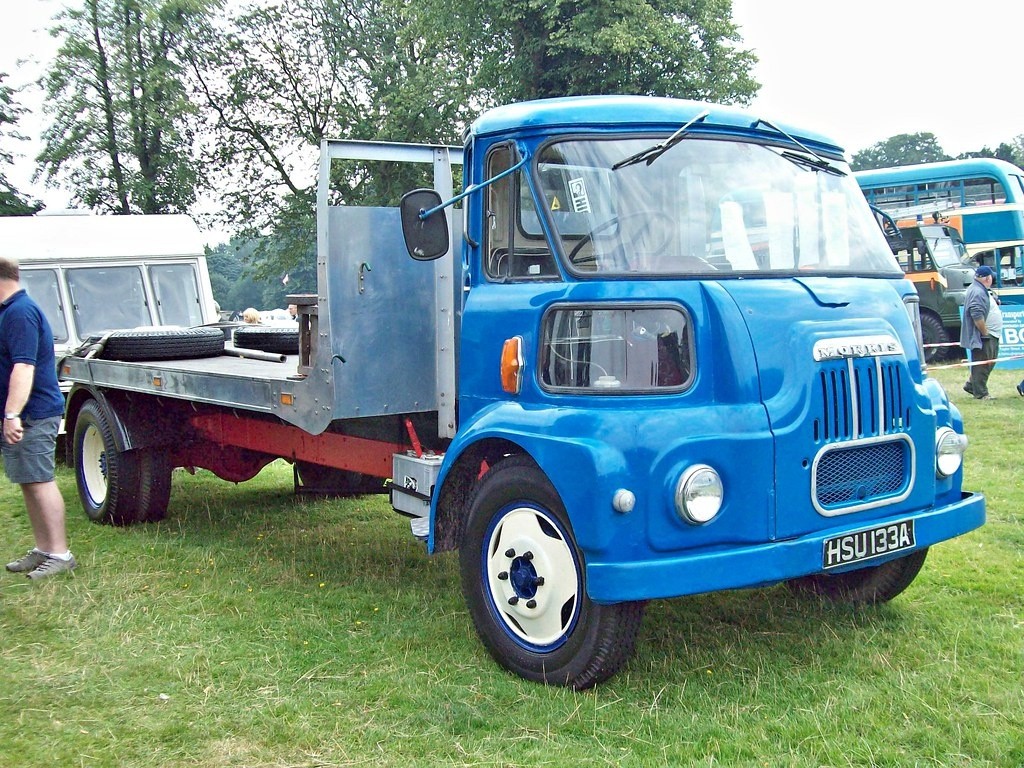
[
  {"x1": 243, "y1": 307, "x2": 262, "y2": 323},
  {"x1": 960, "y1": 266, "x2": 1003, "y2": 399},
  {"x1": 215, "y1": 302, "x2": 231, "y2": 341},
  {"x1": 0, "y1": 256, "x2": 76, "y2": 580},
  {"x1": 289, "y1": 304, "x2": 298, "y2": 321}
]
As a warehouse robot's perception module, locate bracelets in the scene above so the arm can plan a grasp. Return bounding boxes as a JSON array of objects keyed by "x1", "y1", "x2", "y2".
[{"x1": 4, "y1": 414, "x2": 20, "y2": 419}]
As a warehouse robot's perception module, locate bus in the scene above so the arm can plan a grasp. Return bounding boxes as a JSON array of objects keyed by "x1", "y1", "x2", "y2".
[{"x1": 689, "y1": 155, "x2": 1023, "y2": 328}]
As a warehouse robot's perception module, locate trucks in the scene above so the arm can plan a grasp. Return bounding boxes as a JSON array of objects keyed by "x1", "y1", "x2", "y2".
[
  {"x1": 54, "y1": 94, "x2": 988, "y2": 694},
  {"x1": 868, "y1": 201, "x2": 982, "y2": 366}
]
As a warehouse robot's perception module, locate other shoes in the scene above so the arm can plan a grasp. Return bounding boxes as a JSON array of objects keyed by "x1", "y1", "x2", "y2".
[
  {"x1": 1016, "y1": 384, "x2": 1024, "y2": 396},
  {"x1": 963, "y1": 382, "x2": 973, "y2": 394},
  {"x1": 980, "y1": 392, "x2": 996, "y2": 400}
]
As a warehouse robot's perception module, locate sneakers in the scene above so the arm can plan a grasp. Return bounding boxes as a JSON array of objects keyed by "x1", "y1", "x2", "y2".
[
  {"x1": 6, "y1": 550, "x2": 48, "y2": 572},
  {"x1": 26, "y1": 553, "x2": 77, "y2": 579}
]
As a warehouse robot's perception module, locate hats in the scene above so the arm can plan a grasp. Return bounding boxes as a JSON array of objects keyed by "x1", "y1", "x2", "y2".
[{"x1": 975, "y1": 265, "x2": 997, "y2": 278}]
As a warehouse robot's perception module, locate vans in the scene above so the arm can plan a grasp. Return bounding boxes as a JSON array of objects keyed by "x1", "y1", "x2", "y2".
[{"x1": 0, "y1": 213, "x2": 217, "y2": 452}]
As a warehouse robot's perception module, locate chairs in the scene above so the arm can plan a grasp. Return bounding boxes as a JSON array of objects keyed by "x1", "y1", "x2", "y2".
[{"x1": 490, "y1": 247, "x2": 557, "y2": 276}]
